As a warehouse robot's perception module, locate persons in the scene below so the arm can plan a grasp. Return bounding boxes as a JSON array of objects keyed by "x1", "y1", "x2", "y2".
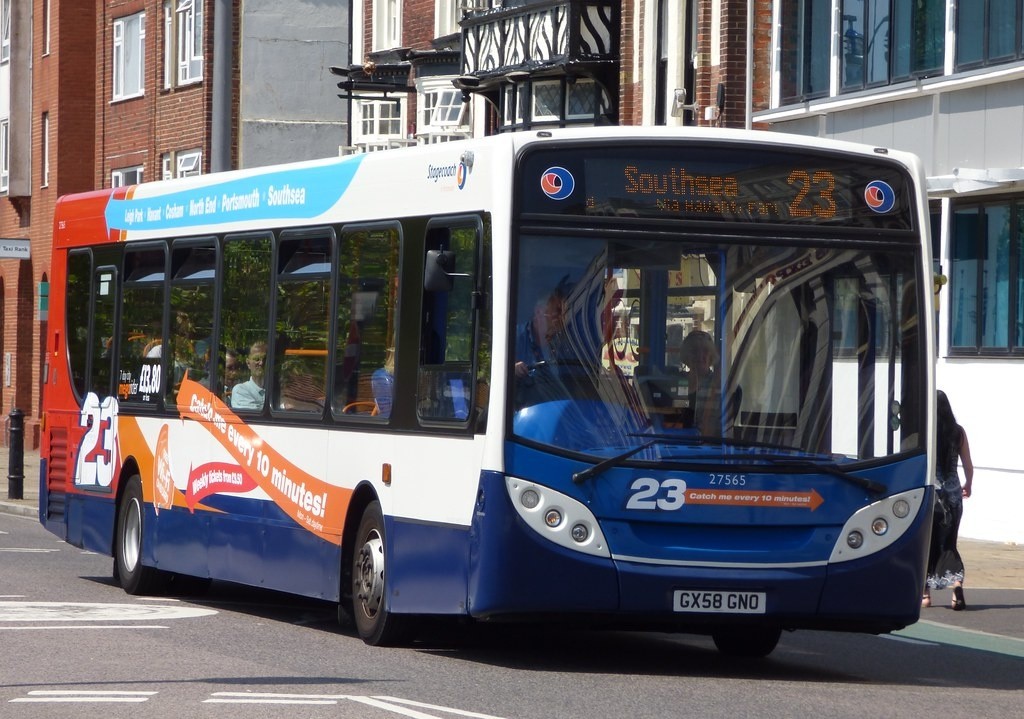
[
  {"x1": 680, "y1": 331, "x2": 742, "y2": 437},
  {"x1": 920, "y1": 391, "x2": 974, "y2": 608},
  {"x1": 147, "y1": 311, "x2": 194, "y2": 405},
  {"x1": 231, "y1": 341, "x2": 266, "y2": 410},
  {"x1": 515, "y1": 296, "x2": 577, "y2": 383},
  {"x1": 372, "y1": 332, "x2": 395, "y2": 418}
]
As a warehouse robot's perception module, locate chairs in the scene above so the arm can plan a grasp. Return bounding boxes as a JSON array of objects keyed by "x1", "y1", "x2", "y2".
[{"x1": 105, "y1": 335, "x2": 378, "y2": 415}]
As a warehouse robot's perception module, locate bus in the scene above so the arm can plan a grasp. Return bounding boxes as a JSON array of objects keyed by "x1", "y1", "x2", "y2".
[{"x1": 34, "y1": 122, "x2": 946, "y2": 665}]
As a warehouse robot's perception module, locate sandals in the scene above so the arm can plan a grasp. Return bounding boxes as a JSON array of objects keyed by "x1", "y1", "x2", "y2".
[
  {"x1": 951, "y1": 585, "x2": 965, "y2": 610},
  {"x1": 921, "y1": 596, "x2": 931, "y2": 608}
]
